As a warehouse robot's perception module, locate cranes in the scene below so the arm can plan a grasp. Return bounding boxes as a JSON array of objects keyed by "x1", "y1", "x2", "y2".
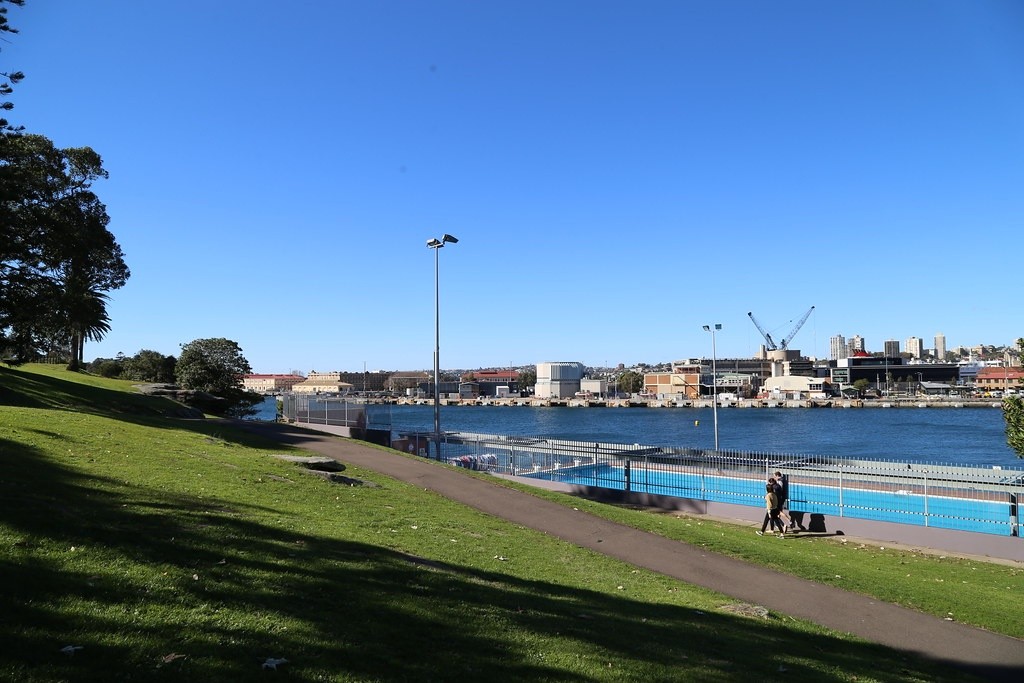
[{"x1": 748, "y1": 306, "x2": 815, "y2": 350}]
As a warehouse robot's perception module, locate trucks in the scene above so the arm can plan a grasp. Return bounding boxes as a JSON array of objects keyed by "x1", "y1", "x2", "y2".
[
  {"x1": 719, "y1": 393, "x2": 743, "y2": 401},
  {"x1": 320, "y1": 391, "x2": 400, "y2": 398}
]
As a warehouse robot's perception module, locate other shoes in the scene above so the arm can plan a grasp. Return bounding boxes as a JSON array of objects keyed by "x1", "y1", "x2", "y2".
[
  {"x1": 776, "y1": 536, "x2": 785, "y2": 539},
  {"x1": 783, "y1": 525, "x2": 787, "y2": 534},
  {"x1": 788, "y1": 522, "x2": 793, "y2": 530},
  {"x1": 766, "y1": 531, "x2": 774, "y2": 534},
  {"x1": 756, "y1": 531, "x2": 763, "y2": 537}
]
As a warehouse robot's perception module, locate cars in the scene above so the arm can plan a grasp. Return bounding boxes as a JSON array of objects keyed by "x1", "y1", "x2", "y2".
[{"x1": 971, "y1": 388, "x2": 1024, "y2": 398}]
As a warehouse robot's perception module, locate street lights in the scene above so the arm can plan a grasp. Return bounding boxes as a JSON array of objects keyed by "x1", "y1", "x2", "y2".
[
  {"x1": 426, "y1": 234, "x2": 460, "y2": 460},
  {"x1": 703, "y1": 323, "x2": 724, "y2": 451},
  {"x1": 915, "y1": 372, "x2": 923, "y2": 382}
]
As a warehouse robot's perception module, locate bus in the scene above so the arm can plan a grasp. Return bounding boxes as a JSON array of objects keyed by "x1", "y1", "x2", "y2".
[
  {"x1": 640, "y1": 394, "x2": 656, "y2": 400},
  {"x1": 575, "y1": 391, "x2": 595, "y2": 400},
  {"x1": 881, "y1": 391, "x2": 908, "y2": 396}
]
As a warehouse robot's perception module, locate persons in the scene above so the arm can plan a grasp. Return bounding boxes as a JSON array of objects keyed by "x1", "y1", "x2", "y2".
[
  {"x1": 755, "y1": 484, "x2": 785, "y2": 539},
  {"x1": 765, "y1": 471, "x2": 793, "y2": 534}
]
